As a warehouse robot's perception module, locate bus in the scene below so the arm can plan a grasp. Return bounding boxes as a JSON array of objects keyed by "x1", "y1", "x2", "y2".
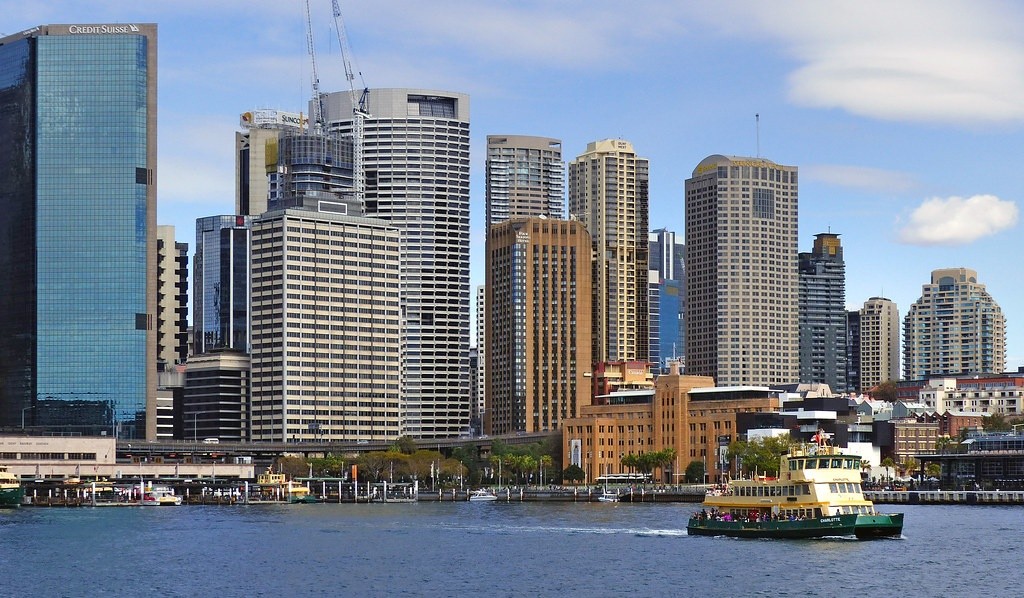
[
  {"x1": 293, "y1": 477, "x2": 345, "y2": 489},
  {"x1": 595, "y1": 476, "x2": 651, "y2": 489},
  {"x1": 601, "y1": 473, "x2": 653, "y2": 483}
]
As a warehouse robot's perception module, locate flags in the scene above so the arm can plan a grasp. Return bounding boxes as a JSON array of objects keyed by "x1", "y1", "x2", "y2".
[{"x1": 815, "y1": 433, "x2": 820, "y2": 443}]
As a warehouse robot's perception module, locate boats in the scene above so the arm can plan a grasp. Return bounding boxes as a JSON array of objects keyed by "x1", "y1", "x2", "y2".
[
  {"x1": 156, "y1": 494, "x2": 182, "y2": 506},
  {"x1": 598, "y1": 490, "x2": 620, "y2": 503},
  {"x1": 470, "y1": 491, "x2": 498, "y2": 501},
  {"x1": 685, "y1": 428, "x2": 904, "y2": 539}
]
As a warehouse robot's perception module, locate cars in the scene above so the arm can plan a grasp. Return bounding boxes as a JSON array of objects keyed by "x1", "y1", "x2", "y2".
[
  {"x1": 202, "y1": 438, "x2": 219, "y2": 445},
  {"x1": 894, "y1": 481, "x2": 907, "y2": 491},
  {"x1": 516, "y1": 431, "x2": 527, "y2": 436},
  {"x1": 478, "y1": 434, "x2": 488, "y2": 440}
]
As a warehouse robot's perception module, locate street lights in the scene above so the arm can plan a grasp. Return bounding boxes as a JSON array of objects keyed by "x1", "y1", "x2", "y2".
[
  {"x1": 22, "y1": 406, "x2": 36, "y2": 430},
  {"x1": 194, "y1": 411, "x2": 206, "y2": 443},
  {"x1": 111, "y1": 407, "x2": 115, "y2": 437}
]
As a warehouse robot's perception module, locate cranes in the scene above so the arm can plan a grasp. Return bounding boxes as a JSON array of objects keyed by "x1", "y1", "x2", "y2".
[
  {"x1": 301, "y1": 1, "x2": 325, "y2": 134},
  {"x1": 328, "y1": 1, "x2": 373, "y2": 214}
]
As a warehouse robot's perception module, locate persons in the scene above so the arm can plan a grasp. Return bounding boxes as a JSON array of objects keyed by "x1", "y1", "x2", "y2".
[
  {"x1": 549, "y1": 483, "x2": 556, "y2": 491},
  {"x1": 651, "y1": 484, "x2": 682, "y2": 492},
  {"x1": 692, "y1": 508, "x2": 807, "y2": 522},
  {"x1": 836, "y1": 509, "x2": 840, "y2": 515},
  {"x1": 705, "y1": 483, "x2": 733, "y2": 496}
]
